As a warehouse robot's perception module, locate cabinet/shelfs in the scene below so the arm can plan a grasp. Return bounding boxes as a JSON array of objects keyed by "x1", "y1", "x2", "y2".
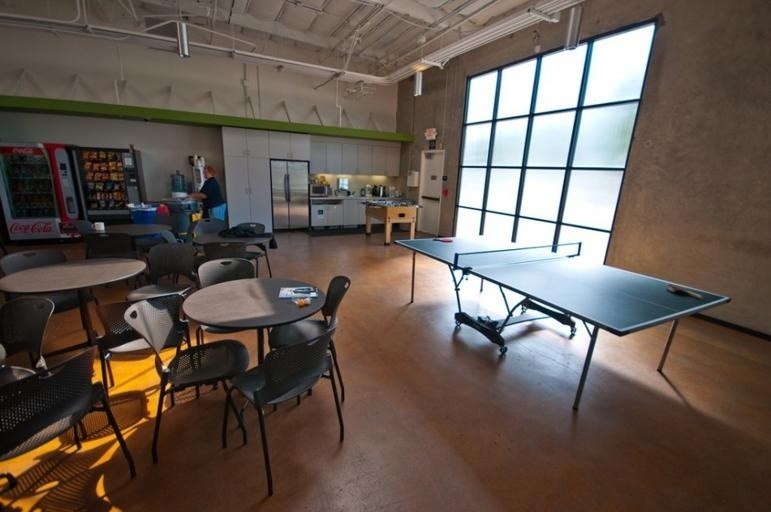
[
  {"x1": 306, "y1": 195, "x2": 405, "y2": 234},
  {"x1": 1, "y1": 143, "x2": 141, "y2": 246},
  {"x1": 172, "y1": 170, "x2": 186, "y2": 192},
  {"x1": 360, "y1": 184, "x2": 386, "y2": 197}
]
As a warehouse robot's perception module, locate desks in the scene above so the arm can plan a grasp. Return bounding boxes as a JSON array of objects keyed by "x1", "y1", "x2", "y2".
[
  {"x1": 393, "y1": 236, "x2": 731, "y2": 411},
  {"x1": 183, "y1": 277, "x2": 327, "y2": 427},
  {"x1": 365, "y1": 199, "x2": 417, "y2": 245}
]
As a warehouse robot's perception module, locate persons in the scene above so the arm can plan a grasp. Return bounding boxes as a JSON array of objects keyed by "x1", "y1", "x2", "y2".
[{"x1": 186, "y1": 164, "x2": 228, "y2": 224}]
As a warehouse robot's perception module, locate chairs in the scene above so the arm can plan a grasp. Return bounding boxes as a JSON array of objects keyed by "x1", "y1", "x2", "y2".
[
  {"x1": 269, "y1": 275, "x2": 350, "y2": 412},
  {"x1": 221, "y1": 315, "x2": 344, "y2": 495},
  {"x1": 0, "y1": 215, "x2": 278, "y2": 512}
]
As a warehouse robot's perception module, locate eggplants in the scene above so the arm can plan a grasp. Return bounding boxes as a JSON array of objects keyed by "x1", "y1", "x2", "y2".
[
  {"x1": 667, "y1": 284, "x2": 701, "y2": 300},
  {"x1": 433, "y1": 238, "x2": 453, "y2": 242}
]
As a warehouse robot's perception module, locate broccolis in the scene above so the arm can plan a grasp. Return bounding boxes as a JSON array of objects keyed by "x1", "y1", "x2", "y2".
[{"x1": 270, "y1": 158, "x2": 311, "y2": 231}]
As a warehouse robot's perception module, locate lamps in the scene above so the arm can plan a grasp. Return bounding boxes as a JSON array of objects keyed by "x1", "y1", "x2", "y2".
[
  {"x1": 565, "y1": 7, "x2": 582, "y2": 51},
  {"x1": 176, "y1": 21, "x2": 191, "y2": 57},
  {"x1": 413, "y1": 71, "x2": 422, "y2": 96}
]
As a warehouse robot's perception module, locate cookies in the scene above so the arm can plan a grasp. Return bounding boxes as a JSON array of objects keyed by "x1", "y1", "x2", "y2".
[{"x1": 308, "y1": 184, "x2": 329, "y2": 197}]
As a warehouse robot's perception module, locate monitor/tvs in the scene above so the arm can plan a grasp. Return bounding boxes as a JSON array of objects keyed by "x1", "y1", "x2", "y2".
[{"x1": 279, "y1": 285, "x2": 320, "y2": 300}]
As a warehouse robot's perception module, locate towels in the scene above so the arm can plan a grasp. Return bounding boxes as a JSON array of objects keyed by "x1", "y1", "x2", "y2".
[{"x1": 129, "y1": 207, "x2": 161, "y2": 251}]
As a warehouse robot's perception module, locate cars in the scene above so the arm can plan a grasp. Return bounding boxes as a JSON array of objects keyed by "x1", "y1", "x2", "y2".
[
  {"x1": 133, "y1": 200, "x2": 151, "y2": 209},
  {"x1": 91, "y1": 222, "x2": 104, "y2": 231},
  {"x1": 157, "y1": 206, "x2": 168, "y2": 215},
  {"x1": 193, "y1": 155, "x2": 205, "y2": 167}
]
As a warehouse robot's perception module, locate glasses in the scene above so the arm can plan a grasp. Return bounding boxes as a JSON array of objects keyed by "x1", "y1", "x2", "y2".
[
  {"x1": 413, "y1": 71, "x2": 422, "y2": 96},
  {"x1": 176, "y1": 21, "x2": 191, "y2": 57},
  {"x1": 565, "y1": 7, "x2": 582, "y2": 51}
]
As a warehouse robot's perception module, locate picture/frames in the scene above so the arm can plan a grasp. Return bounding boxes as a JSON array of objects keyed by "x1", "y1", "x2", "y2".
[
  {"x1": 393, "y1": 236, "x2": 731, "y2": 411},
  {"x1": 365, "y1": 199, "x2": 417, "y2": 245}
]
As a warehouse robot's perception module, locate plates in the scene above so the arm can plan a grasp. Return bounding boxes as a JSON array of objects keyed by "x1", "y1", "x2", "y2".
[
  {"x1": 1, "y1": 143, "x2": 141, "y2": 246},
  {"x1": 306, "y1": 195, "x2": 405, "y2": 234}
]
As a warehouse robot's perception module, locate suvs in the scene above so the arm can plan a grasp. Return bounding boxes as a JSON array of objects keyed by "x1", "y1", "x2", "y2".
[{"x1": 170, "y1": 173, "x2": 185, "y2": 192}]
